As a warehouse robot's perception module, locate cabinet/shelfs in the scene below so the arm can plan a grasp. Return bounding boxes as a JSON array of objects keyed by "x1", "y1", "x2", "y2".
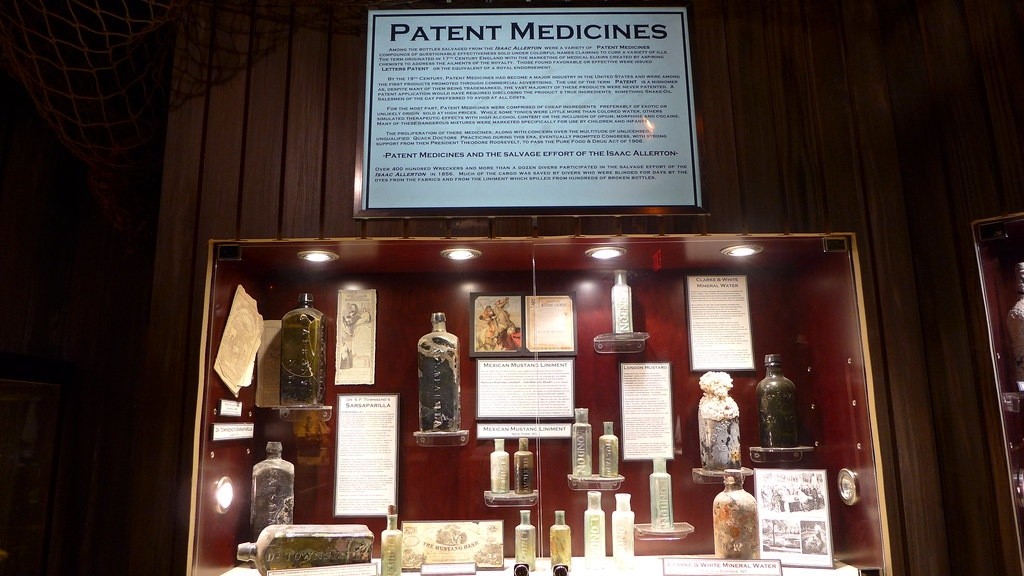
[
  {"x1": 185, "y1": 233, "x2": 890, "y2": 576},
  {"x1": 971, "y1": 211, "x2": 1024, "y2": 576}
]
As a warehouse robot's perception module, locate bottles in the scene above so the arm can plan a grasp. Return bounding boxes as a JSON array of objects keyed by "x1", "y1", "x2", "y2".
[
  {"x1": 250, "y1": 441, "x2": 295, "y2": 569},
  {"x1": 610, "y1": 269, "x2": 633, "y2": 334},
  {"x1": 380, "y1": 505, "x2": 403, "y2": 576},
  {"x1": 490, "y1": 438, "x2": 510, "y2": 494},
  {"x1": 699, "y1": 371, "x2": 742, "y2": 470},
  {"x1": 573, "y1": 407, "x2": 592, "y2": 477},
  {"x1": 417, "y1": 312, "x2": 462, "y2": 433},
  {"x1": 513, "y1": 437, "x2": 533, "y2": 494},
  {"x1": 515, "y1": 491, "x2": 635, "y2": 569},
  {"x1": 649, "y1": 458, "x2": 674, "y2": 530},
  {"x1": 756, "y1": 354, "x2": 799, "y2": 448},
  {"x1": 280, "y1": 293, "x2": 326, "y2": 406},
  {"x1": 599, "y1": 422, "x2": 618, "y2": 478},
  {"x1": 237, "y1": 524, "x2": 375, "y2": 576},
  {"x1": 713, "y1": 468, "x2": 760, "y2": 561}
]
